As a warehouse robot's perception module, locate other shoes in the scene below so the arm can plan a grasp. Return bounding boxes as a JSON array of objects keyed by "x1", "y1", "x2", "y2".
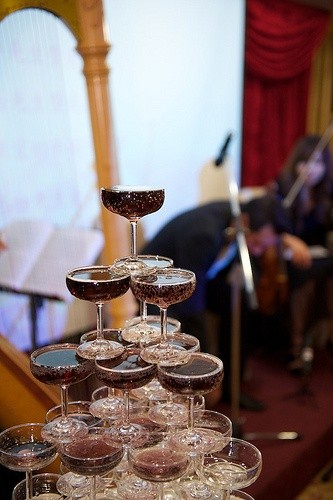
[
  {"x1": 290, "y1": 355, "x2": 306, "y2": 379},
  {"x1": 226, "y1": 392, "x2": 268, "y2": 413}
]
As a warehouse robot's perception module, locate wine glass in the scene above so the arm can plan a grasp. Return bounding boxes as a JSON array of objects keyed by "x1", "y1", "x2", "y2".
[{"x1": 0, "y1": 185, "x2": 262, "y2": 500}]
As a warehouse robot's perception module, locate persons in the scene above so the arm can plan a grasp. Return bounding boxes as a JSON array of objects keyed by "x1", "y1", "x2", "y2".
[
  {"x1": 141, "y1": 196, "x2": 289, "y2": 412},
  {"x1": 271, "y1": 135, "x2": 333, "y2": 377}
]
